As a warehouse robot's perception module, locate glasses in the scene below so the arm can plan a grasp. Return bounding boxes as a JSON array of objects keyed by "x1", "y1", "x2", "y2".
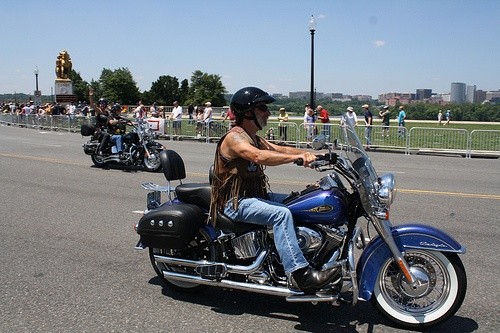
[{"x1": 99, "y1": 103, "x2": 107, "y2": 105}]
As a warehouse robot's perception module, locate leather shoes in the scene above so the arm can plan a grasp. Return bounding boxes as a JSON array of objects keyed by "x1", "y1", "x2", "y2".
[{"x1": 293, "y1": 266, "x2": 341, "y2": 295}]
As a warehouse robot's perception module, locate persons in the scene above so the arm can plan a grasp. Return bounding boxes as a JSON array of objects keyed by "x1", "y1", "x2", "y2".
[
  {"x1": 222, "y1": 107, "x2": 236, "y2": 131},
  {"x1": 361, "y1": 104, "x2": 372, "y2": 145},
  {"x1": 341, "y1": 106, "x2": 357, "y2": 145},
  {"x1": 188, "y1": 101, "x2": 213, "y2": 137},
  {"x1": 133, "y1": 101, "x2": 163, "y2": 122},
  {"x1": 89, "y1": 90, "x2": 133, "y2": 159},
  {"x1": 316, "y1": 105, "x2": 330, "y2": 142},
  {"x1": 304, "y1": 104, "x2": 314, "y2": 148},
  {"x1": 171, "y1": 101, "x2": 182, "y2": 135},
  {"x1": 437, "y1": 109, "x2": 451, "y2": 126},
  {"x1": 208, "y1": 87, "x2": 341, "y2": 294},
  {"x1": 0, "y1": 101, "x2": 88, "y2": 131},
  {"x1": 378, "y1": 106, "x2": 390, "y2": 136},
  {"x1": 278, "y1": 107, "x2": 288, "y2": 143},
  {"x1": 398, "y1": 105, "x2": 406, "y2": 137}
]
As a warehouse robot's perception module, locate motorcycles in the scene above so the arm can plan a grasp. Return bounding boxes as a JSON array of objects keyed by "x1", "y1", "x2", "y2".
[
  {"x1": 132, "y1": 110, "x2": 468, "y2": 328},
  {"x1": 80, "y1": 115, "x2": 164, "y2": 173}
]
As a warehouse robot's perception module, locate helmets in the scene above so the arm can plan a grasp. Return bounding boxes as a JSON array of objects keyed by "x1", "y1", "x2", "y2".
[
  {"x1": 98, "y1": 98, "x2": 107, "y2": 105},
  {"x1": 230, "y1": 87, "x2": 276, "y2": 116},
  {"x1": 111, "y1": 104, "x2": 121, "y2": 111}
]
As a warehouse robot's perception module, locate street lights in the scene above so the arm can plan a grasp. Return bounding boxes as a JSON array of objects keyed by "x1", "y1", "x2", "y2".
[
  {"x1": 33, "y1": 66, "x2": 39, "y2": 91},
  {"x1": 307, "y1": 15, "x2": 317, "y2": 123}
]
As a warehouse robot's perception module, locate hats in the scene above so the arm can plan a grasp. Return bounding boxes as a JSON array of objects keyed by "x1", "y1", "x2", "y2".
[
  {"x1": 280, "y1": 108, "x2": 285, "y2": 110},
  {"x1": 205, "y1": 102, "x2": 212, "y2": 105},
  {"x1": 347, "y1": 107, "x2": 353, "y2": 112},
  {"x1": 362, "y1": 104, "x2": 368, "y2": 108}
]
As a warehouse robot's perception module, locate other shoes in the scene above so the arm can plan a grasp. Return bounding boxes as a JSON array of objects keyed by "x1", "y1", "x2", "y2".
[
  {"x1": 99, "y1": 150, "x2": 103, "y2": 156},
  {"x1": 119, "y1": 154, "x2": 126, "y2": 160}
]
